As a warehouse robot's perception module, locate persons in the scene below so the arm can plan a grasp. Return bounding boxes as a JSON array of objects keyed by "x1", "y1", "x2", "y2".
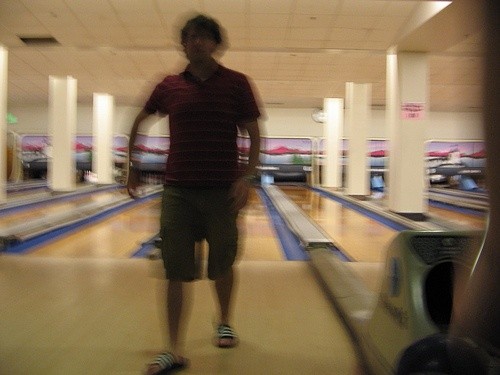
[
  {"x1": 394, "y1": 330, "x2": 500, "y2": 375},
  {"x1": 128, "y1": 14, "x2": 261, "y2": 375}
]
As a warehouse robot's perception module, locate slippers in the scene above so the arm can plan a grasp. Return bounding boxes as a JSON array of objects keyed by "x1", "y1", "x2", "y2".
[
  {"x1": 211, "y1": 318, "x2": 239, "y2": 348},
  {"x1": 144, "y1": 351, "x2": 190, "y2": 375}
]
